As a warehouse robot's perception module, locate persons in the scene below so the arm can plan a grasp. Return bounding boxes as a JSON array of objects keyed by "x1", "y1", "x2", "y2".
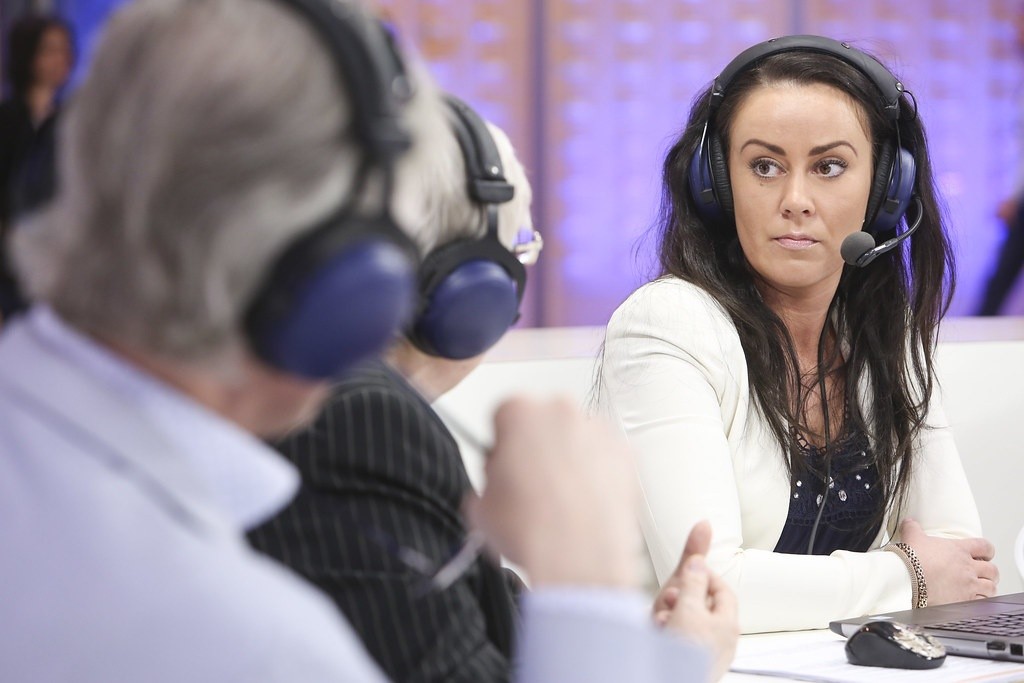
[
  {"x1": 0, "y1": 0, "x2": 733, "y2": 683},
  {"x1": 594, "y1": 33, "x2": 1000, "y2": 631},
  {"x1": 977, "y1": 180, "x2": 1024, "y2": 317}
]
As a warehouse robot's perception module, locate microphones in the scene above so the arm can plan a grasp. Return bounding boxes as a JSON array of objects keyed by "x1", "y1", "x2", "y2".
[{"x1": 840, "y1": 197, "x2": 924, "y2": 267}]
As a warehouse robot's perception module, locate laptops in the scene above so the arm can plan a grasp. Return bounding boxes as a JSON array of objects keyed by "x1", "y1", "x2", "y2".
[{"x1": 829, "y1": 591, "x2": 1024, "y2": 663}]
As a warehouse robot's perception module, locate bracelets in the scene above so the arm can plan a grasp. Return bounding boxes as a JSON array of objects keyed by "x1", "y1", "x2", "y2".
[{"x1": 884, "y1": 539, "x2": 928, "y2": 610}]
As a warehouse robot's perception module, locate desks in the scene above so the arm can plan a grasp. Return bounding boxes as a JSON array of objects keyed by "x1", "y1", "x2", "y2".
[{"x1": 720, "y1": 628, "x2": 1024, "y2": 683}]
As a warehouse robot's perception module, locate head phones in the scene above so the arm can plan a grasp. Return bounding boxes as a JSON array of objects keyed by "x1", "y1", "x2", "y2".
[
  {"x1": 398, "y1": 97, "x2": 527, "y2": 361},
  {"x1": 684, "y1": 35, "x2": 917, "y2": 229},
  {"x1": 32, "y1": 0, "x2": 427, "y2": 376}
]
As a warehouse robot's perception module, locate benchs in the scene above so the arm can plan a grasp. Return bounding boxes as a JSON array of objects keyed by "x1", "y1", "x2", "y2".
[{"x1": 427, "y1": 316, "x2": 1024, "y2": 598}]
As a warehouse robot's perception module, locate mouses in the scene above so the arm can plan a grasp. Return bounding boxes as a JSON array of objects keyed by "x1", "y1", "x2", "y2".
[{"x1": 843, "y1": 621, "x2": 947, "y2": 671}]
